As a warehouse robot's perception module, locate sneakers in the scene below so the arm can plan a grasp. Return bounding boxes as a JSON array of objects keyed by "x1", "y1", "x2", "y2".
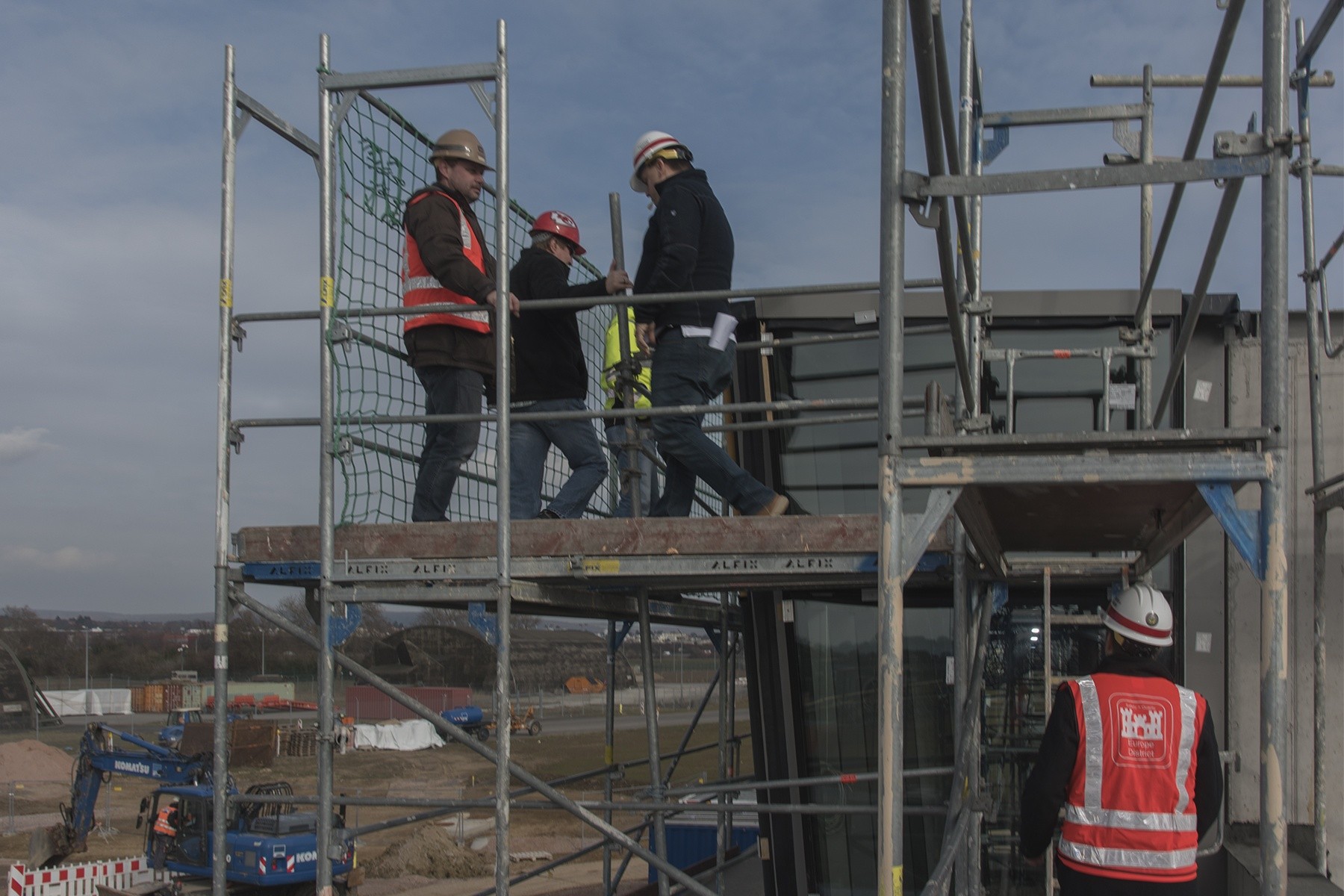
[{"x1": 755, "y1": 494, "x2": 789, "y2": 516}]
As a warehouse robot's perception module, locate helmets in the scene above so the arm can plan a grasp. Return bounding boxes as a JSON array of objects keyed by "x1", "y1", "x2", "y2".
[
  {"x1": 1103, "y1": 584, "x2": 1176, "y2": 649},
  {"x1": 629, "y1": 130, "x2": 680, "y2": 194},
  {"x1": 429, "y1": 130, "x2": 496, "y2": 175},
  {"x1": 528, "y1": 209, "x2": 587, "y2": 257},
  {"x1": 174, "y1": 796, "x2": 180, "y2": 802}
]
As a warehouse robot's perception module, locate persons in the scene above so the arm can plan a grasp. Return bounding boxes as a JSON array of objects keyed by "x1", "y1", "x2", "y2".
[
  {"x1": 601, "y1": 302, "x2": 658, "y2": 516},
  {"x1": 629, "y1": 131, "x2": 794, "y2": 517},
  {"x1": 402, "y1": 129, "x2": 522, "y2": 521},
  {"x1": 1018, "y1": 583, "x2": 1222, "y2": 896},
  {"x1": 508, "y1": 210, "x2": 634, "y2": 519}
]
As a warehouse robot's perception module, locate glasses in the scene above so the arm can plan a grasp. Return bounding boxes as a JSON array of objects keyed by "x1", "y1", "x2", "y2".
[{"x1": 564, "y1": 243, "x2": 576, "y2": 258}]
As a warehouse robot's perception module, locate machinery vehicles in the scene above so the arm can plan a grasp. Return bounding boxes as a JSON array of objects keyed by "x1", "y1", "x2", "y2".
[
  {"x1": 432, "y1": 706, "x2": 490, "y2": 743},
  {"x1": 37, "y1": 722, "x2": 354, "y2": 896},
  {"x1": 509, "y1": 706, "x2": 542, "y2": 737}
]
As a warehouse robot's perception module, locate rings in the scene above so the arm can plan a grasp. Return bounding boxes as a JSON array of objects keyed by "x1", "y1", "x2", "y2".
[{"x1": 623, "y1": 278, "x2": 626, "y2": 284}]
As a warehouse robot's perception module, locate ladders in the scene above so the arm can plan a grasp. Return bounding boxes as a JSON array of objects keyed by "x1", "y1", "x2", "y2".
[{"x1": 1043, "y1": 565, "x2": 1130, "y2": 896}]
